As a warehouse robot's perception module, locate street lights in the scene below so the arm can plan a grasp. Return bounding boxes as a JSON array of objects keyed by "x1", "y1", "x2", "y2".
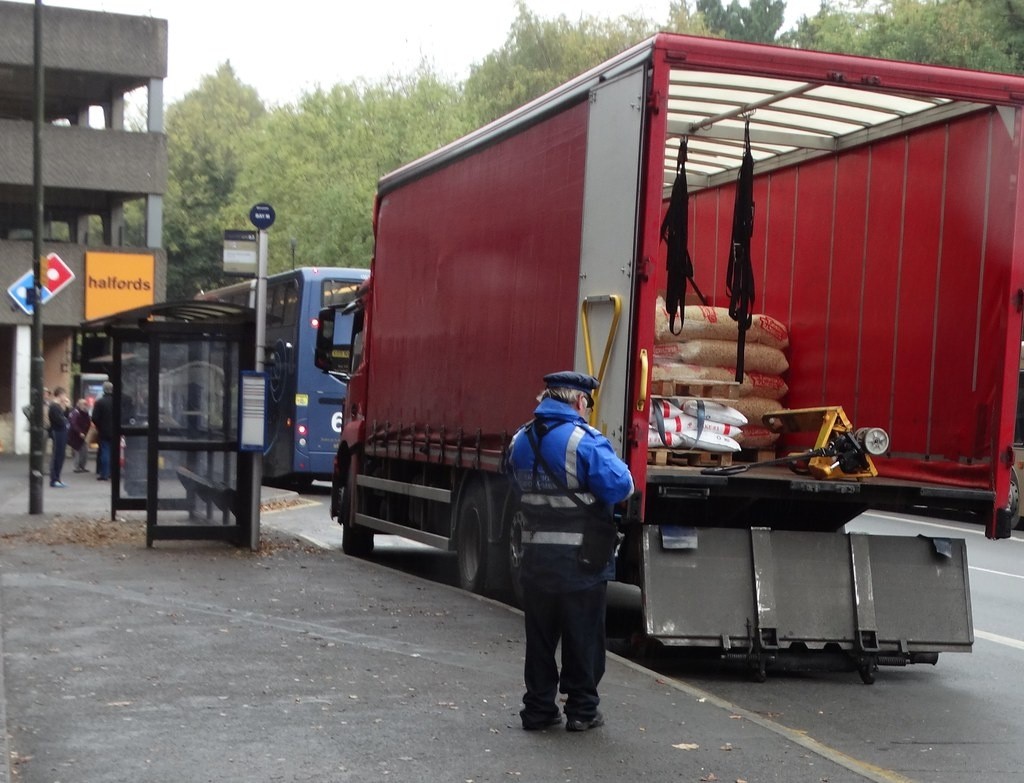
[{"x1": 289, "y1": 236, "x2": 298, "y2": 270}]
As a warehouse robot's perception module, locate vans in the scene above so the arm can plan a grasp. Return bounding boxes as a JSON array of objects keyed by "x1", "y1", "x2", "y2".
[{"x1": 874, "y1": 339, "x2": 1024, "y2": 532}]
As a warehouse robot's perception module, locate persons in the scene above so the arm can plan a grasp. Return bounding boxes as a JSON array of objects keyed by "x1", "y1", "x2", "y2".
[
  {"x1": 69, "y1": 399, "x2": 91, "y2": 473},
  {"x1": 48, "y1": 387, "x2": 71, "y2": 488},
  {"x1": 91, "y1": 382, "x2": 134, "y2": 481},
  {"x1": 24, "y1": 388, "x2": 51, "y2": 475},
  {"x1": 504, "y1": 371, "x2": 635, "y2": 731}
]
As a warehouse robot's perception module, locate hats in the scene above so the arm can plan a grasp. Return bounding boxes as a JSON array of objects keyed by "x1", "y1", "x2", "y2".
[
  {"x1": 102, "y1": 381, "x2": 114, "y2": 394},
  {"x1": 544, "y1": 373, "x2": 599, "y2": 396},
  {"x1": 43, "y1": 387, "x2": 51, "y2": 395}
]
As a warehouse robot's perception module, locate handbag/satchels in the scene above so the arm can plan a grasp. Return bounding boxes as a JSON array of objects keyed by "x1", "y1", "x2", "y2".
[{"x1": 577, "y1": 515, "x2": 616, "y2": 575}]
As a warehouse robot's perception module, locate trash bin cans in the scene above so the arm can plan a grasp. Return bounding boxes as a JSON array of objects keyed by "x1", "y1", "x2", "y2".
[{"x1": 124, "y1": 420, "x2": 159, "y2": 497}]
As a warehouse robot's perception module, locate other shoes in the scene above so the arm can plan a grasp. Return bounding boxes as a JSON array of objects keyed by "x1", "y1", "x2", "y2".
[
  {"x1": 73, "y1": 467, "x2": 88, "y2": 473},
  {"x1": 50, "y1": 480, "x2": 66, "y2": 487},
  {"x1": 97, "y1": 476, "x2": 110, "y2": 480}
]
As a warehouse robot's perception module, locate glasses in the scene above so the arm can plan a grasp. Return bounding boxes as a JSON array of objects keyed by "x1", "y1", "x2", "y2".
[{"x1": 583, "y1": 396, "x2": 593, "y2": 415}]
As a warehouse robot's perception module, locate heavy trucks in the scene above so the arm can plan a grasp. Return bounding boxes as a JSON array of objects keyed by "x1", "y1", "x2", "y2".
[{"x1": 310, "y1": 27, "x2": 1024, "y2": 673}]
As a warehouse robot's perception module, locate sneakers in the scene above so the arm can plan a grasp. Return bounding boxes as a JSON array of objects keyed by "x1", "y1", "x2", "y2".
[
  {"x1": 566, "y1": 709, "x2": 604, "y2": 732},
  {"x1": 522, "y1": 708, "x2": 562, "y2": 729}
]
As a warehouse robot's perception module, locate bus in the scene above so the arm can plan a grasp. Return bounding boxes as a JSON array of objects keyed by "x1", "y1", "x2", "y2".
[
  {"x1": 81, "y1": 372, "x2": 109, "y2": 406},
  {"x1": 187, "y1": 264, "x2": 373, "y2": 492}
]
